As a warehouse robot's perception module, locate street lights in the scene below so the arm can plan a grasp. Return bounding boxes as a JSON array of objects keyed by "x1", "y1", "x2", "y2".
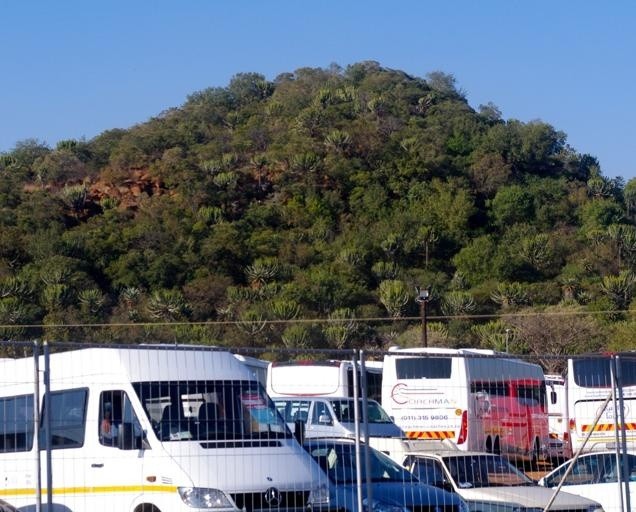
[{"x1": 416, "y1": 285, "x2": 431, "y2": 350}]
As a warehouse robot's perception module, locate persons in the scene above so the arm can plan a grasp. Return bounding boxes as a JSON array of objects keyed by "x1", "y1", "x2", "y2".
[{"x1": 99, "y1": 396, "x2": 120, "y2": 440}]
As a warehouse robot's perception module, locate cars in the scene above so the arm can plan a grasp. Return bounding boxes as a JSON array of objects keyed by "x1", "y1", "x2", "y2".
[
  {"x1": 537, "y1": 448, "x2": 636, "y2": 512},
  {"x1": 302, "y1": 435, "x2": 469, "y2": 512}
]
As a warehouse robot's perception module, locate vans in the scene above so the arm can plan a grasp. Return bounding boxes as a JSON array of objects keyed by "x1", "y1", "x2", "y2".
[{"x1": 270, "y1": 395, "x2": 406, "y2": 440}]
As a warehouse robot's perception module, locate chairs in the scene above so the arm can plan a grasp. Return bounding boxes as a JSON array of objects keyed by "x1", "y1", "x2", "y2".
[
  {"x1": 279, "y1": 408, "x2": 307, "y2": 422},
  {"x1": 158, "y1": 402, "x2": 228, "y2": 432}
]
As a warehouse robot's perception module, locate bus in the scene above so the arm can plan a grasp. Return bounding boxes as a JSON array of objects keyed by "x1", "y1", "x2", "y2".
[
  {"x1": 566, "y1": 350, "x2": 636, "y2": 458},
  {"x1": 543, "y1": 375, "x2": 572, "y2": 460},
  {"x1": 264, "y1": 345, "x2": 384, "y2": 415},
  {"x1": 2, "y1": 342, "x2": 334, "y2": 512},
  {"x1": 383, "y1": 346, "x2": 549, "y2": 469}
]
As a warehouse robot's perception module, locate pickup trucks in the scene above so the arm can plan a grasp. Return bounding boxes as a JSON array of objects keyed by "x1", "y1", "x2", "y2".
[{"x1": 327, "y1": 439, "x2": 605, "y2": 512}]
